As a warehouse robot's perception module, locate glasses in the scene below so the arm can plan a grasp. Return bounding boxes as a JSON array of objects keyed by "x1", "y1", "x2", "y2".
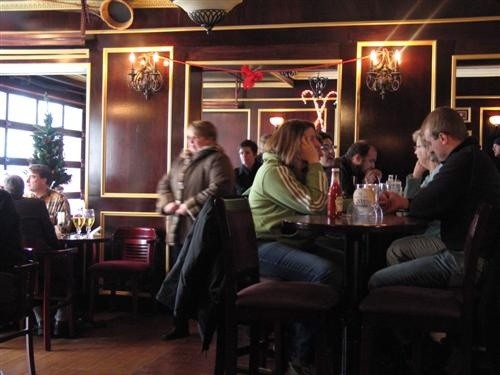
[
  {"x1": 414, "y1": 145, "x2": 426, "y2": 149},
  {"x1": 320, "y1": 145, "x2": 337, "y2": 152}
]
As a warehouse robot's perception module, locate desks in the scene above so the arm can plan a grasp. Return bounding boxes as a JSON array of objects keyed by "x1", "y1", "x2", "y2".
[
  {"x1": 57, "y1": 232, "x2": 112, "y2": 329},
  {"x1": 279, "y1": 215, "x2": 426, "y2": 375}
]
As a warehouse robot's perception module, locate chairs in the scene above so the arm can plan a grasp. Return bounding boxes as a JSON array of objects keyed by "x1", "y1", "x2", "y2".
[
  {"x1": 209, "y1": 195, "x2": 338, "y2": 375},
  {"x1": 357, "y1": 198, "x2": 495, "y2": 375},
  {"x1": 0, "y1": 247, "x2": 79, "y2": 375},
  {"x1": 87, "y1": 226, "x2": 160, "y2": 322}
]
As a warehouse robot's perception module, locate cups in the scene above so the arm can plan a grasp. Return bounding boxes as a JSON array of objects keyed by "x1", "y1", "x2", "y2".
[{"x1": 352, "y1": 181, "x2": 403, "y2": 225}]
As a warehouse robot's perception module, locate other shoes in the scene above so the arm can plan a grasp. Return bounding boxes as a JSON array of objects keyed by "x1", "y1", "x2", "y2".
[
  {"x1": 55, "y1": 319, "x2": 83, "y2": 336},
  {"x1": 162, "y1": 327, "x2": 189, "y2": 340},
  {"x1": 289, "y1": 358, "x2": 314, "y2": 375},
  {"x1": 38, "y1": 319, "x2": 55, "y2": 336}
]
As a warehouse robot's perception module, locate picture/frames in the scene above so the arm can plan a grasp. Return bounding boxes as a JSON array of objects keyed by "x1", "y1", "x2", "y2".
[{"x1": 456, "y1": 106, "x2": 471, "y2": 123}]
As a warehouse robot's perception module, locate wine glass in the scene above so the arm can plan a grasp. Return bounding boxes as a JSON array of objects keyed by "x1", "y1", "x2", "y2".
[
  {"x1": 83, "y1": 208, "x2": 96, "y2": 239},
  {"x1": 72, "y1": 212, "x2": 86, "y2": 238}
]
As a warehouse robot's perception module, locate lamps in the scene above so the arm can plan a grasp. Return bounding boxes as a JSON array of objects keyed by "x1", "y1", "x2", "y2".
[
  {"x1": 364, "y1": 48, "x2": 403, "y2": 100},
  {"x1": 128, "y1": 51, "x2": 162, "y2": 101},
  {"x1": 169, "y1": 0, "x2": 246, "y2": 37},
  {"x1": 269, "y1": 116, "x2": 284, "y2": 130}
]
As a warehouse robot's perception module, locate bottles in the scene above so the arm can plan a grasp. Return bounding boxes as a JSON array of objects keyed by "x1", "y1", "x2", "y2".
[{"x1": 326, "y1": 168, "x2": 344, "y2": 220}]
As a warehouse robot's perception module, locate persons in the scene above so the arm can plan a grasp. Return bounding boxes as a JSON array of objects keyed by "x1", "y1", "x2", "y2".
[
  {"x1": 3, "y1": 174, "x2": 80, "y2": 336},
  {"x1": 369, "y1": 107, "x2": 500, "y2": 375},
  {"x1": 248, "y1": 119, "x2": 358, "y2": 375},
  {"x1": 302, "y1": 131, "x2": 335, "y2": 167},
  {"x1": 26, "y1": 164, "x2": 71, "y2": 225},
  {"x1": 492, "y1": 135, "x2": 500, "y2": 158},
  {"x1": 255, "y1": 134, "x2": 274, "y2": 163},
  {"x1": 387, "y1": 128, "x2": 447, "y2": 264},
  {"x1": 0, "y1": 189, "x2": 24, "y2": 333},
  {"x1": 233, "y1": 140, "x2": 263, "y2": 198},
  {"x1": 323, "y1": 140, "x2": 382, "y2": 198},
  {"x1": 157, "y1": 120, "x2": 235, "y2": 340}
]
